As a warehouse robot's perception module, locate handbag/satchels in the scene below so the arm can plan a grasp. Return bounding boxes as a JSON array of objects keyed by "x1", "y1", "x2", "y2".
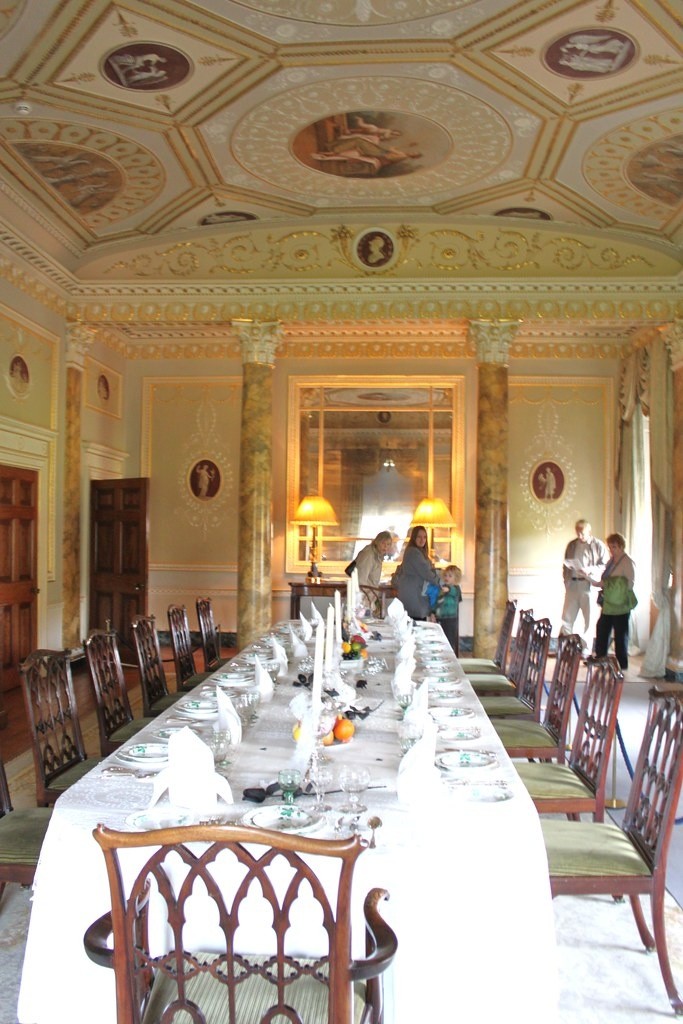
[
  {"x1": 391, "y1": 564, "x2": 403, "y2": 591},
  {"x1": 344, "y1": 560, "x2": 356, "y2": 578}
]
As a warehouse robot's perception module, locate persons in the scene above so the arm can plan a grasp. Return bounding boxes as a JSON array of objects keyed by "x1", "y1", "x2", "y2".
[
  {"x1": 584, "y1": 533, "x2": 637, "y2": 672},
  {"x1": 400, "y1": 525, "x2": 441, "y2": 622},
  {"x1": 352, "y1": 531, "x2": 392, "y2": 616},
  {"x1": 440, "y1": 565, "x2": 463, "y2": 652},
  {"x1": 557, "y1": 519, "x2": 610, "y2": 663}
]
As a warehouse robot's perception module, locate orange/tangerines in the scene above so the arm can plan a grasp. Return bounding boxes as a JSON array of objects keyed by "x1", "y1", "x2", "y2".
[
  {"x1": 342, "y1": 642, "x2": 368, "y2": 658},
  {"x1": 292, "y1": 715, "x2": 354, "y2": 744}
]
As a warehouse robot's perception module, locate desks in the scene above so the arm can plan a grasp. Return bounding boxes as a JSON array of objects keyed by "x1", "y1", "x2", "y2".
[{"x1": 289, "y1": 579, "x2": 394, "y2": 620}]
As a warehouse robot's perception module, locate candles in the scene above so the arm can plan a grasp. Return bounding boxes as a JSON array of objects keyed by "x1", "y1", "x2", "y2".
[{"x1": 297, "y1": 567, "x2": 362, "y2": 780}]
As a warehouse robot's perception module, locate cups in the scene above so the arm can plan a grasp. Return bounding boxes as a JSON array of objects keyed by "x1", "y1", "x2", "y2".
[{"x1": 397, "y1": 722, "x2": 422, "y2": 757}]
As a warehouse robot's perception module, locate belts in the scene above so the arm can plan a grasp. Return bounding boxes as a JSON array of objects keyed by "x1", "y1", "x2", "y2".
[{"x1": 572, "y1": 577, "x2": 586, "y2": 582}]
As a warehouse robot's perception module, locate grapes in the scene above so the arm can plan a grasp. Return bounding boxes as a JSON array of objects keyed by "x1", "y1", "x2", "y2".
[{"x1": 342, "y1": 650, "x2": 358, "y2": 660}]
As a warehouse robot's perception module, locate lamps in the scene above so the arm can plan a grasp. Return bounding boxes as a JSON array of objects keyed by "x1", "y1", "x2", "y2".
[
  {"x1": 412, "y1": 497, "x2": 458, "y2": 562},
  {"x1": 289, "y1": 495, "x2": 339, "y2": 581}
]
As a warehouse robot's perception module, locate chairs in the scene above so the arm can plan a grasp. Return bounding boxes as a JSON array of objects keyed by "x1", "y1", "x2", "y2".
[
  {"x1": 456, "y1": 601, "x2": 682, "y2": 1012},
  {"x1": 0, "y1": 599, "x2": 231, "y2": 896},
  {"x1": 81, "y1": 824, "x2": 399, "y2": 1024}
]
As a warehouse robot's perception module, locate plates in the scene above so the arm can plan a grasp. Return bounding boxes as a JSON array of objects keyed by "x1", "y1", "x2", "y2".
[{"x1": 100, "y1": 616, "x2": 512, "y2": 838}]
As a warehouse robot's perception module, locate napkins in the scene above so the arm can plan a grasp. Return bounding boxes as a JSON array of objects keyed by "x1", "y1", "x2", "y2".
[
  {"x1": 388, "y1": 598, "x2": 437, "y2": 784},
  {"x1": 150, "y1": 600, "x2": 322, "y2": 817}
]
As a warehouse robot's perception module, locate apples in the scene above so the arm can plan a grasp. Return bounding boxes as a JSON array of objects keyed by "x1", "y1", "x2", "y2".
[{"x1": 350, "y1": 635, "x2": 365, "y2": 644}]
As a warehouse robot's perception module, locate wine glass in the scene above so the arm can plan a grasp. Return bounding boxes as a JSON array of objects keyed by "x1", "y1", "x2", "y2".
[
  {"x1": 209, "y1": 739, "x2": 229, "y2": 772},
  {"x1": 278, "y1": 769, "x2": 301, "y2": 806},
  {"x1": 310, "y1": 765, "x2": 332, "y2": 813},
  {"x1": 338, "y1": 765, "x2": 371, "y2": 813},
  {"x1": 305, "y1": 692, "x2": 336, "y2": 764},
  {"x1": 214, "y1": 730, "x2": 231, "y2": 766}
]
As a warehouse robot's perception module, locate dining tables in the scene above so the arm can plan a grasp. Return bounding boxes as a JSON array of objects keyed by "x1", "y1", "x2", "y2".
[{"x1": 20, "y1": 618, "x2": 549, "y2": 1024}]
